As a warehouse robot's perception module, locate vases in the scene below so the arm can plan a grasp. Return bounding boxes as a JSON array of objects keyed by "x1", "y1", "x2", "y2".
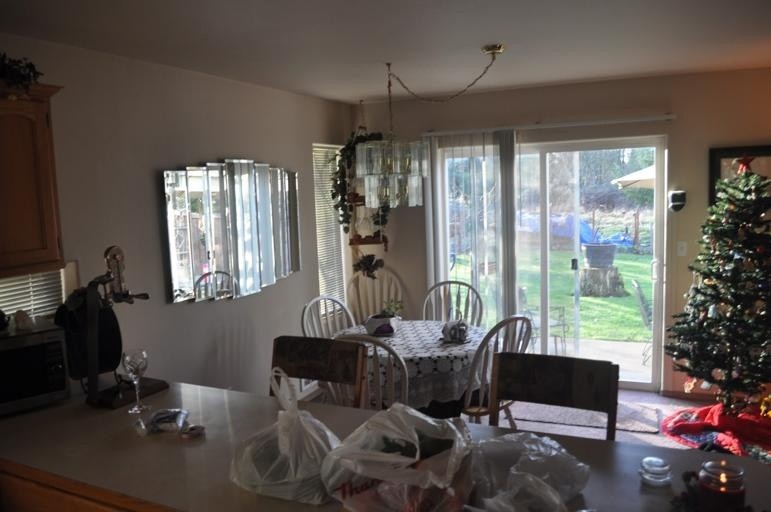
[{"x1": 580, "y1": 242, "x2": 617, "y2": 269}]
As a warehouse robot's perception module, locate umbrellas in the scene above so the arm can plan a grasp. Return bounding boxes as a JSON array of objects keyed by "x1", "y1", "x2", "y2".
[{"x1": 610, "y1": 163, "x2": 655, "y2": 190}]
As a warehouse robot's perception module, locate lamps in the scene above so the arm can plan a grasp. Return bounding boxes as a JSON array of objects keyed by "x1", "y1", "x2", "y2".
[{"x1": 356, "y1": 44, "x2": 508, "y2": 211}]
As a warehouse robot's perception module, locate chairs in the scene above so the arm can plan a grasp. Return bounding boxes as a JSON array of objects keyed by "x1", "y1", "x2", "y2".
[
  {"x1": 624, "y1": 277, "x2": 655, "y2": 366},
  {"x1": 487, "y1": 347, "x2": 621, "y2": 440},
  {"x1": 299, "y1": 264, "x2": 533, "y2": 428},
  {"x1": 268, "y1": 334, "x2": 367, "y2": 412},
  {"x1": 193, "y1": 268, "x2": 241, "y2": 302},
  {"x1": 516, "y1": 285, "x2": 571, "y2": 355}
]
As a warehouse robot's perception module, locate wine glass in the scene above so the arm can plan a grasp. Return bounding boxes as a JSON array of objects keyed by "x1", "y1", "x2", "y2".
[{"x1": 122, "y1": 349, "x2": 153, "y2": 414}]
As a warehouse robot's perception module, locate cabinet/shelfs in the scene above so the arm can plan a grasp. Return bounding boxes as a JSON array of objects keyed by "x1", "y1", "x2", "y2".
[{"x1": 2, "y1": 79, "x2": 69, "y2": 283}]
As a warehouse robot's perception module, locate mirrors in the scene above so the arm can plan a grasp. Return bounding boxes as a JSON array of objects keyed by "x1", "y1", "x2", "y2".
[{"x1": 161, "y1": 156, "x2": 304, "y2": 306}]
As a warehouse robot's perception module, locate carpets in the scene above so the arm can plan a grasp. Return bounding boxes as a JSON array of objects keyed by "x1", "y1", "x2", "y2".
[{"x1": 506, "y1": 396, "x2": 663, "y2": 436}]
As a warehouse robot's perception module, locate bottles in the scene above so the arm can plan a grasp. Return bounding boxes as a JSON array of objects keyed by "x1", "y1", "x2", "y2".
[{"x1": 696, "y1": 459, "x2": 746, "y2": 512}]
